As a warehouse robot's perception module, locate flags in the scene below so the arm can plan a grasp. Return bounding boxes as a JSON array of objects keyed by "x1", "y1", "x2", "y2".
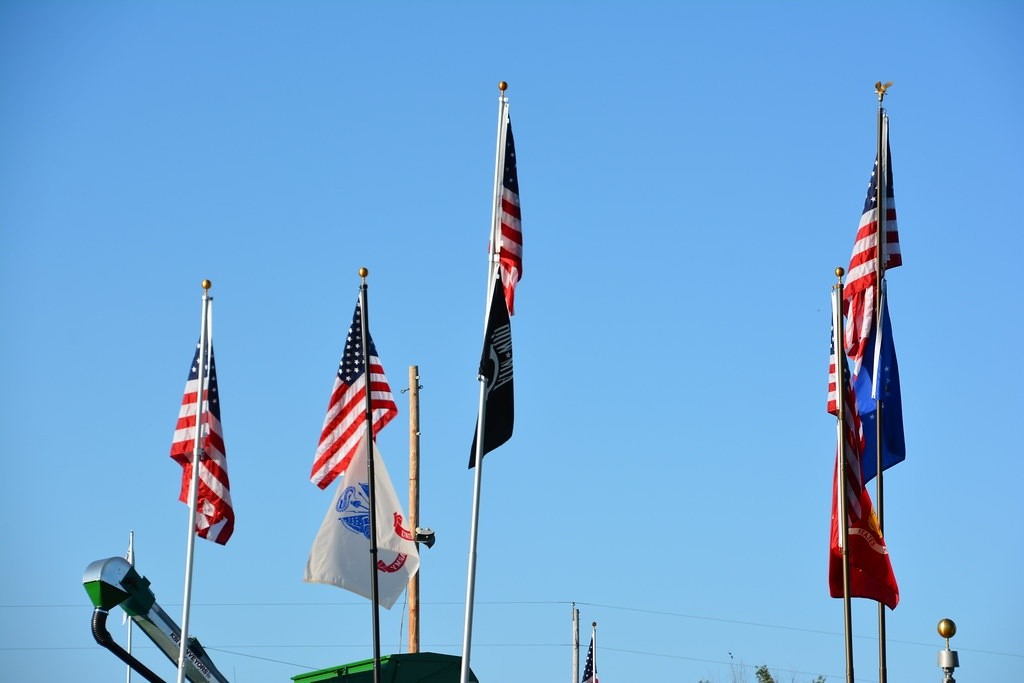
[
  {"x1": 467, "y1": 102, "x2": 523, "y2": 470},
  {"x1": 581, "y1": 628, "x2": 599, "y2": 683},
  {"x1": 309, "y1": 279, "x2": 397, "y2": 491},
  {"x1": 303, "y1": 430, "x2": 419, "y2": 610},
  {"x1": 170, "y1": 296, "x2": 235, "y2": 546},
  {"x1": 827, "y1": 109, "x2": 907, "y2": 612}
]
